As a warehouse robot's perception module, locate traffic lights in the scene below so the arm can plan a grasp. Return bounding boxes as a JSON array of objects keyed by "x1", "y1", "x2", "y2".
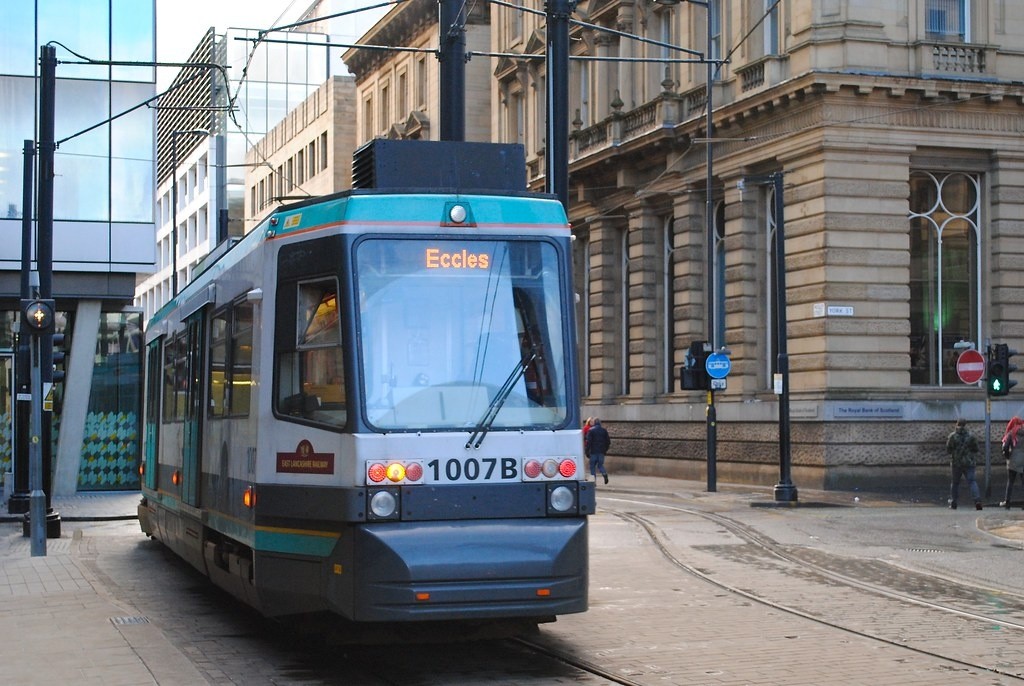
[
  {"x1": 1000, "y1": 344, "x2": 1019, "y2": 393},
  {"x1": 40, "y1": 326, "x2": 68, "y2": 386},
  {"x1": 986, "y1": 347, "x2": 1007, "y2": 396},
  {"x1": 19, "y1": 298, "x2": 58, "y2": 336}
]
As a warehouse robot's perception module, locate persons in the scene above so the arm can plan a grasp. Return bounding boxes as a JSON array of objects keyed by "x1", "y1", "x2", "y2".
[
  {"x1": 999, "y1": 416, "x2": 1024, "y2": 510},
  {"x1": 583, "y1": 416, "x2": 611, "y2": 487},
  {"x1": 946, "y1": 416, "x2": 983, "y2": 510}
]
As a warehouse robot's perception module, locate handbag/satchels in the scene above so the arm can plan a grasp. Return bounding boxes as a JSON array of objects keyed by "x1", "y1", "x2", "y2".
[{"x1": 1004, "y1": 435, "x2": 1012, "y2": 459}]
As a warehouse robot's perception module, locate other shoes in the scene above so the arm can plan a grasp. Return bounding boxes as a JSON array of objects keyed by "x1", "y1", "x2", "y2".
[
  {"x1": 976, "y1": 502, "x2": 983, "y2": 510},
  {"x1": 603, "y1": 473, "x2": 608, "y2": 484},
  {"x1": 999, "y1": 501, "x2": 1010, "y2": 509},
  {"x1": 951, "y1": 501, "x2": 957, "y2": 509}
]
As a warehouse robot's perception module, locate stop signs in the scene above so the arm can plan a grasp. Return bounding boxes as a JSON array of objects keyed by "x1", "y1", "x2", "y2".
[{"x1": 956, "y1": 350, "x2": 985, "y2": 384}]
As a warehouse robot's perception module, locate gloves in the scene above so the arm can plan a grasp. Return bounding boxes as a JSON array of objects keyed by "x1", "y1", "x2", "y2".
[{"x1": 586, "y1": 448, "x2": 591, "y2": 458}]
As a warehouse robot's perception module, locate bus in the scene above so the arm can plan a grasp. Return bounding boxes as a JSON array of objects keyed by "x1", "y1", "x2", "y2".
[{"x1": 128, "y1": 135, "x2": 598, "y2": 647}]
